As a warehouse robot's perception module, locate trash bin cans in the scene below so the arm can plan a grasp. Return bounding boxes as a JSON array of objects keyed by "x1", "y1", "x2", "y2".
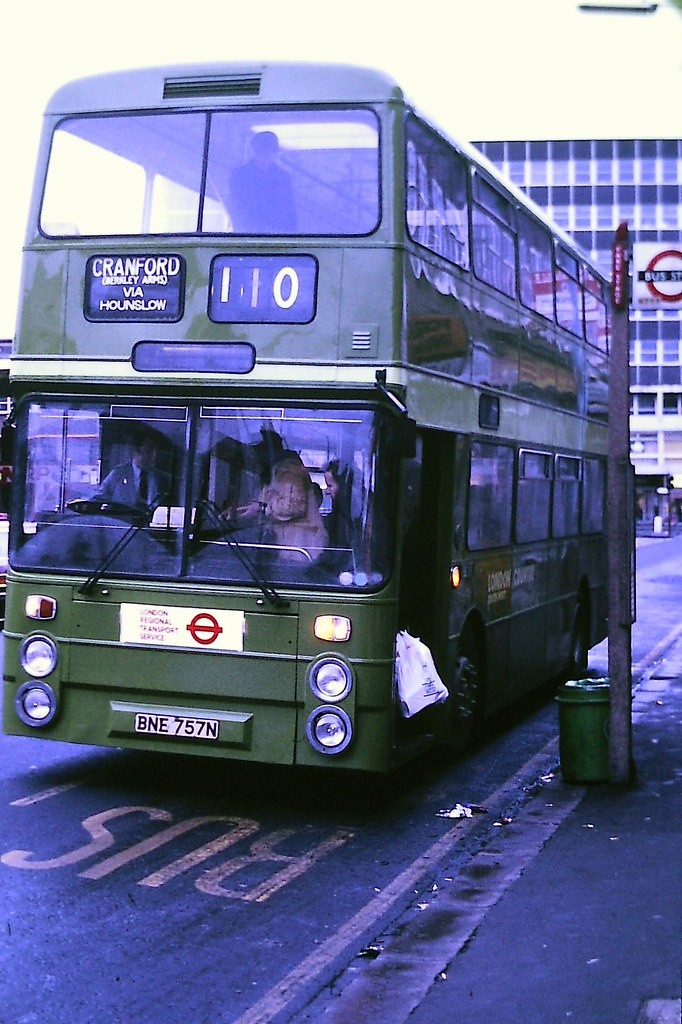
[{"x1": 555, "y1": 676, "x2": 616, "y2": 784}]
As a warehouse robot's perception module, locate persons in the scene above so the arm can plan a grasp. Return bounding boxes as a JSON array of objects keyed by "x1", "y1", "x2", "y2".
[
  {"x1": 456, "y1": 485, "x2": 503, "y2": 549},
  {"x1": 65, "y1": 432, "x2": 366, "y2": 564},
  {"x1": 222, "y1": 132, "x2": 298, "y2": 233}
]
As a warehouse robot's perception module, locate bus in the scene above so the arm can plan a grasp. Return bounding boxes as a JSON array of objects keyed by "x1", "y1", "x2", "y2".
[{"x1": 3, "y1": 63, "x2": 636, "y2": 789}]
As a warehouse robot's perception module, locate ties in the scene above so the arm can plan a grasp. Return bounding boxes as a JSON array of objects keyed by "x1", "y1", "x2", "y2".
[{"x1": 136, "y1": 470, "x2": 148, "y2": 512}]
